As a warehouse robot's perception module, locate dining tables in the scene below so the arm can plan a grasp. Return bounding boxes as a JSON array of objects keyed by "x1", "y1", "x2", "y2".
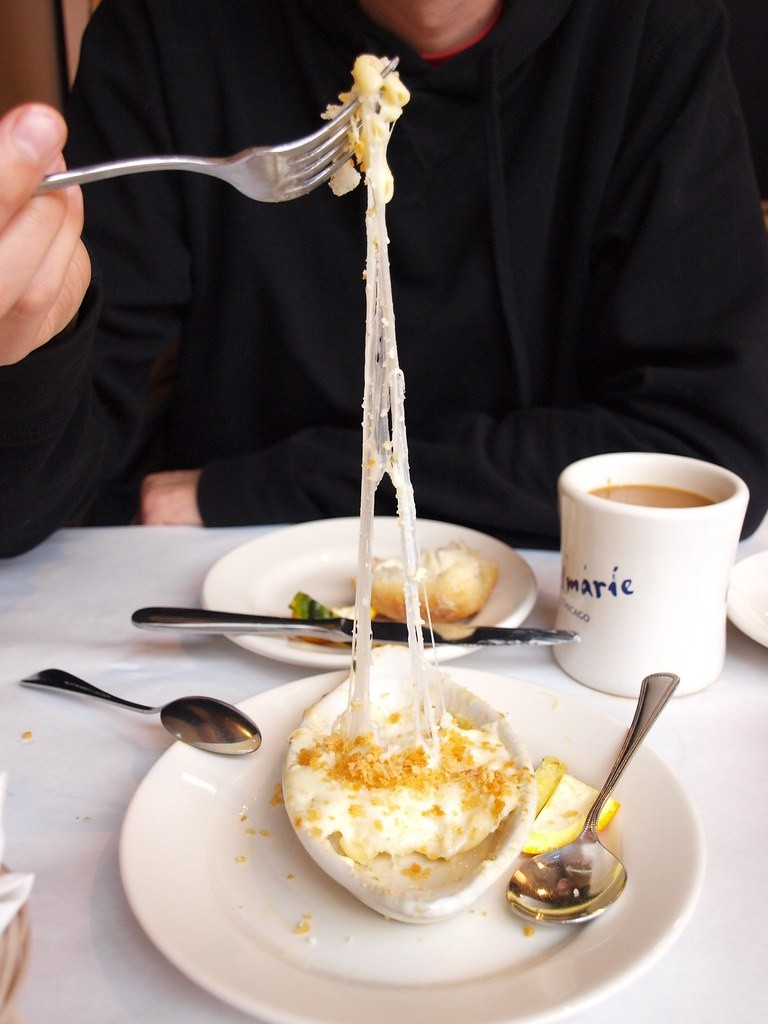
[{"x1": 0, "y1": 524, "x2": 768, "y2": 1024}]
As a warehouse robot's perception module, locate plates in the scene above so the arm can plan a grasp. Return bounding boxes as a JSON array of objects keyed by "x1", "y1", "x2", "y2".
[
  {"x1": 118, "y1": 660, "x2": 703, "y2": 1024},
  {"x1": 726, "y1": 548, "x2": 768, "y2": 651},
  {"x1": 199, "y1": 515, "x2": 538, "y2": 669},
  {"x1": 285, "y1": 641, "x2": 532, "y2": 922}
]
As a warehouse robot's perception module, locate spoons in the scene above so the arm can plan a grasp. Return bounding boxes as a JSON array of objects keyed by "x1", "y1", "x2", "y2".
[
  {"x1": 506, "y1": 671, "x2": 685, "y2": 927},
  {"x1": 20, "y1": 669, "x2": 262, "y2": 757}
]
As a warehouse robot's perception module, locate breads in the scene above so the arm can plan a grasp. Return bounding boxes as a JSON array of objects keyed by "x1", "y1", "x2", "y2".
[{"x1": 352, "y1": 543, "x2": 501, "y2": 623}]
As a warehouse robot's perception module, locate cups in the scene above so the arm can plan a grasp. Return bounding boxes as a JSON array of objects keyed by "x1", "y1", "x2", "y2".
[{"x1": 550, "y1": 452, "x2": 749, "y2": 697}]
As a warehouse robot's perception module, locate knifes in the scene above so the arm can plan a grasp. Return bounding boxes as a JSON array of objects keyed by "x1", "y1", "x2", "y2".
[{"x1": 131, "y1": 605, "x2": 580, "y2": 650}]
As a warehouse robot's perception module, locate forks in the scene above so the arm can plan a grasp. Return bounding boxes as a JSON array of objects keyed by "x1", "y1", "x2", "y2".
[{"x1": 33, "y1": 58, "x2": 399, "y2": 205}]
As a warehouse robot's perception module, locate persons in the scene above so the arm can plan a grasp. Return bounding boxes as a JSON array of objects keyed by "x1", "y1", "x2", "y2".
[{"x1": 1, "y1": 0, "x2": 768, "y2": 562}]
